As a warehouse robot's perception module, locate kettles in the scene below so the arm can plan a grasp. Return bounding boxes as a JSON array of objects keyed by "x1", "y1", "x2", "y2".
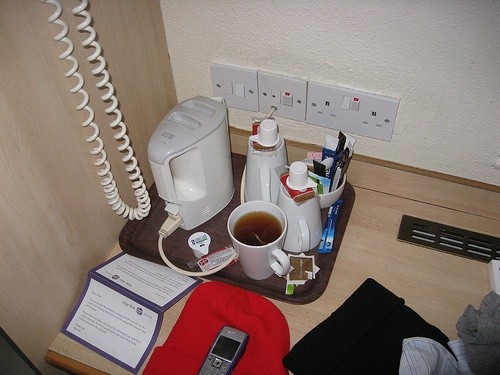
[{"x1": 145, "y1": 95, "x2": 236, "y2": 237}]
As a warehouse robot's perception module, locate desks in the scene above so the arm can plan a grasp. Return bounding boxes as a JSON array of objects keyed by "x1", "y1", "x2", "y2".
[{"x1": 44, "y1": 185, "x2": 500, "y2": 375}]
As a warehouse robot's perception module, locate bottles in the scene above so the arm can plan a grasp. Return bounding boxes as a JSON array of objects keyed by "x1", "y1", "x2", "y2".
[
  {"x1": 278, "y1": 162, "x2": 324, "y2": 252},
  {"x1": 243, "y1": 120, "x2": 287, "y2": 202}
]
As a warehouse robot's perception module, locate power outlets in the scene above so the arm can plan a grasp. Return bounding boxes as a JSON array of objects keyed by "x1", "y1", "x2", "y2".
[
  {"x1": 258, "y1": 71, "x2": 308, "y2": 122},
  {"x1": 306, "y1": 80, "x2": 401, "y2": 142},
  {"x1": 210, "y1": 63, "x2": 258, "y2": 113}
]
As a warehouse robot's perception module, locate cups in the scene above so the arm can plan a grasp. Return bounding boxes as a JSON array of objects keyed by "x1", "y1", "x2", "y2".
[{"x1": 227, "y1": 200, "x2": 290, "y2": 281}]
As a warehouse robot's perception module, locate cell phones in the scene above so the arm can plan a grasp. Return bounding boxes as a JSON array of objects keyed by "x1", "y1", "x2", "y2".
[{"x1": 199, "y1": 326, "x2": 249, "y2": 375}]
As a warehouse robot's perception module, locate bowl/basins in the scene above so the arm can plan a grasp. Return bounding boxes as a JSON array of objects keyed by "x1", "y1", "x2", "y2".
[{"x1": 301, "y1": 158, "x2": 346, "y2": 209}]
